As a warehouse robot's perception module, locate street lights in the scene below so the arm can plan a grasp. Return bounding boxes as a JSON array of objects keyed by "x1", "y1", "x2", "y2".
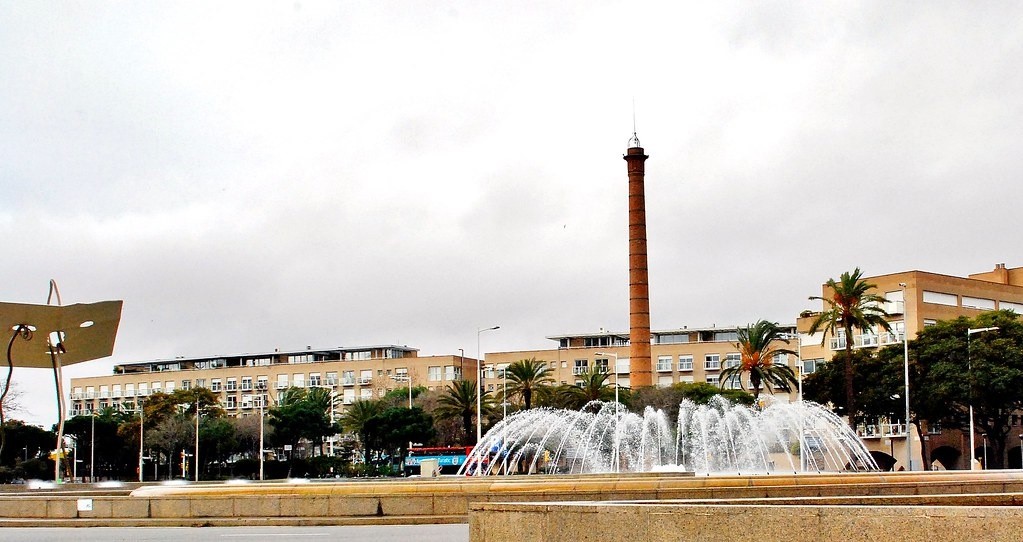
[
  {"x1": 967, "y1": 325, "x2": 1001, "y2": 471},
  {"x1": 775, "y1": 331, "x2": 805, "y2": 473},
  {"x1": 391, "y1": 376, "x2": 413, "y2": 449},
  {"x1": 476, "y1": 325, "x2": 501, "y2": 478},
  {"x1": 595, "y1": 352, "x2": 619, "y2": 473}
]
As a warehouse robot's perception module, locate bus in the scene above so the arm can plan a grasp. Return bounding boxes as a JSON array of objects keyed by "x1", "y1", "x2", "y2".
[{"x1": 405, "y1": 445, "x2": 490, "y2": 477}]
